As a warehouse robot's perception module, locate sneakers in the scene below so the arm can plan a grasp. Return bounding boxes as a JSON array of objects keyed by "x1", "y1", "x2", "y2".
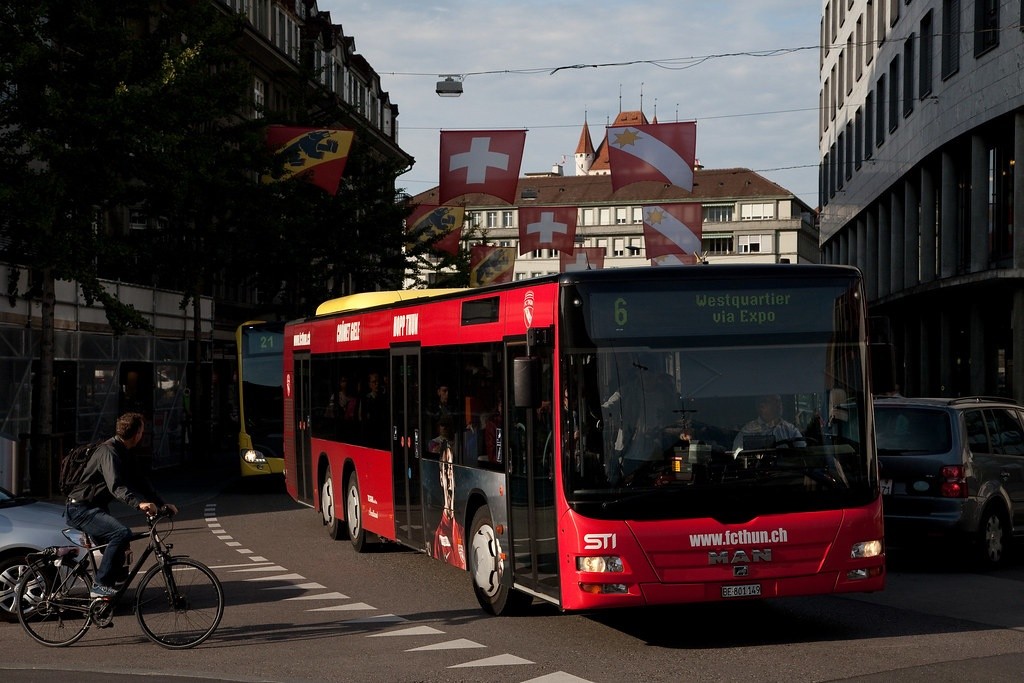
[{"x1": 90, "y1": 582, "x2": 119, "y2": 599}]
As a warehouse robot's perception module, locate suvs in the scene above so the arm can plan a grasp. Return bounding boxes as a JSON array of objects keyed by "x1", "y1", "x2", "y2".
[{"x1": 873, "y1": 392, "x2": 1024, "y2": 575}]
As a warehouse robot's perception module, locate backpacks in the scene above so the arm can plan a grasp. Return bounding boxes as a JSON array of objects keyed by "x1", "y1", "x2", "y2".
[{"x1": 58, "y1": 440, "x2": 122, "y2": 498}]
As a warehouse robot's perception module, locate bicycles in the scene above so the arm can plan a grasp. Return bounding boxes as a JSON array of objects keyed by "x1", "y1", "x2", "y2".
[{"x1": 16, "y1": 500, "x2": 224, "y2": 650}]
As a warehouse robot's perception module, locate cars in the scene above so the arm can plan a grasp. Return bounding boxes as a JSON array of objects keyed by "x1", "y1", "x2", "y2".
[{"x1": 0, "y1": 488, "x2": 132, "y2": 619}]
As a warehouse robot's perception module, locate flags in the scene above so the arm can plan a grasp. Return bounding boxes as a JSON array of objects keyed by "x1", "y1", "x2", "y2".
[
  {"x1": 517, "y1": 208, "x2": 580, "y2": 256},
  {"x1": 604, "y1": 122, "x2": 698, "y2": 194},
  {"x1": 468, "y1": 246, "x2": 517, "y2": 288},
  {"x1": 640, "y1": 202, "x2": 703, "y2": 260},
  {"x1": 266, "y1": 126, "x2": 354, "y2": 197},
  {"x1": 438, "y1": 130, "x2": 529, "y2": 207},
  {"x1": 404, "y1": 204, "x2": 465, "y2": 259},
  {"x1": 556, "y1": 246, "x2": 605, "y2": 274},
  {"x1": 650, "y1": 252, "x2": 698, "y2": 266}
]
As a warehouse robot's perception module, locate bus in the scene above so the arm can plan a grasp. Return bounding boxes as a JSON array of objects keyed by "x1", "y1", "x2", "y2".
[
  {"x1": 233, "y1": 313, "x2": 297, "y2": 489},
  {"x1": 282, "y1": 256, "x2": 888, "y2": 618}
]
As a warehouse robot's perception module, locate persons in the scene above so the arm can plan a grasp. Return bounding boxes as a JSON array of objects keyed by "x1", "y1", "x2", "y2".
[
  {"x1": 594, "y1": 353, "x2": 680, "y2": 485},
  {"x1": 310, "y1": 336, "x2": 589, "y2": 500},
  {"x1": 430, "y1": 445, "x2": 467, "y2": 570},
  {"x1": 731, "y1": 390, "x2": 807, "y2": 460},
  {"x1": 64, "y1": 412, "x2": 178, "y2": 600}
]
